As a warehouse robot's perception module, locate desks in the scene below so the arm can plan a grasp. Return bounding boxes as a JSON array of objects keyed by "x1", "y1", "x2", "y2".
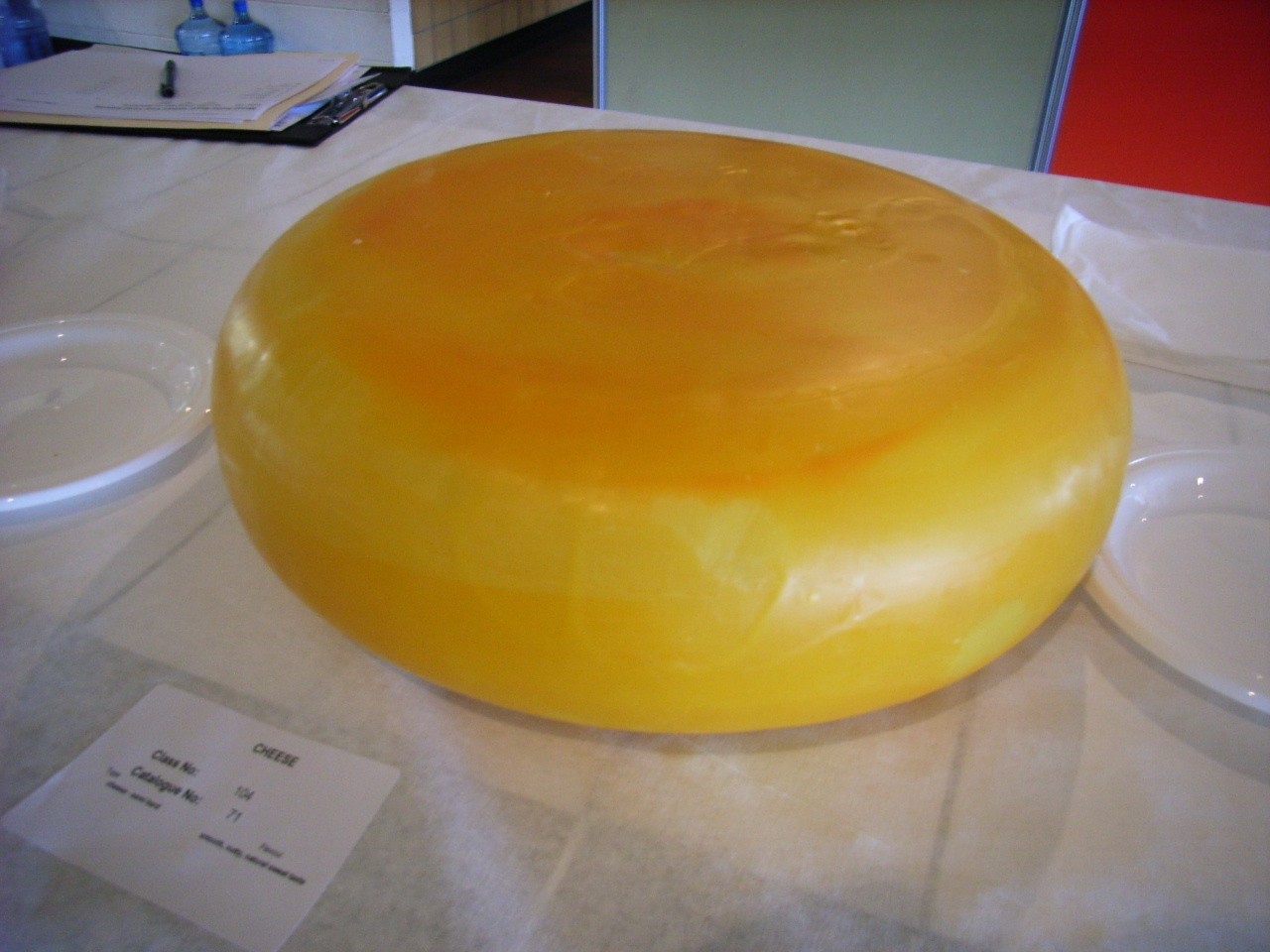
[{"x1": 0, "y1": 88, "x2": 1270, "y2": 952}]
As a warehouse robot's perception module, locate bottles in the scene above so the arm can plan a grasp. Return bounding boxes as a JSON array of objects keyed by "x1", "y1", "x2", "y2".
[
  {"x1": 173, "y1": 0, "x2": 226, "y2": 56},
  {"x1": 218, "y1": 0, "x2": 274, "y2": 56},
  {"x1": 0, "y1": 0, "x2": 55, "y2": 68}
]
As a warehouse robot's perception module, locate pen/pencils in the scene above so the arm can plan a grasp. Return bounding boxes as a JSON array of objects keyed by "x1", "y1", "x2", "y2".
[{"x1": 161, "y1": 60, "x2": 175, "y2": 97}]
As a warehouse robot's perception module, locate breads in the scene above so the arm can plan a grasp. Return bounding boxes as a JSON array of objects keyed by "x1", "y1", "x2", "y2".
[{"x1": 205, "y1": 129, "x2": 1128, "y2": 734}]
